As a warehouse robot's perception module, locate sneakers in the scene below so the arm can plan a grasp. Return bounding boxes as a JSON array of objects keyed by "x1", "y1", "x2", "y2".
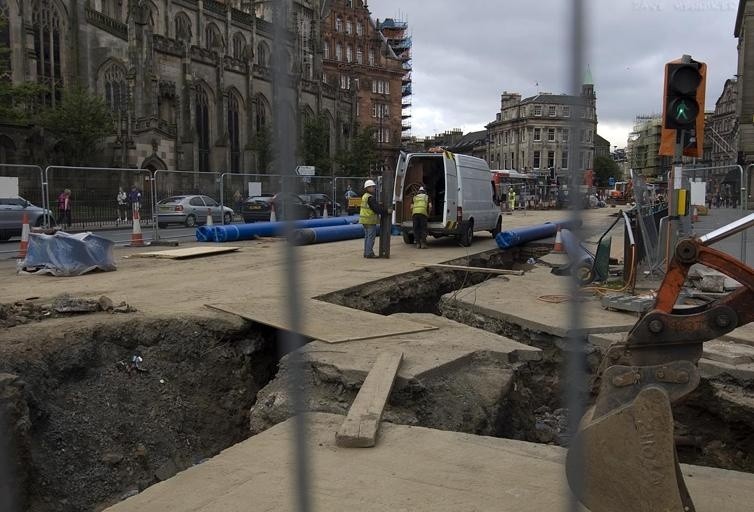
[{"x1": 364, "y1": 254, "x2": 379, "y2": 258}]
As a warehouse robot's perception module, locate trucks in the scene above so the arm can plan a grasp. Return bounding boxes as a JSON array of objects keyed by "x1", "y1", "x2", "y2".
[{"x1": 389, "y1": 147, "x2": 504, "y2": 247}]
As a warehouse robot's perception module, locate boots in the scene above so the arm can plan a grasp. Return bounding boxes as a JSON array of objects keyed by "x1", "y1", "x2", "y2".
[{"x1": 416, "y1": 240, "x2": 428, "y2": 248}]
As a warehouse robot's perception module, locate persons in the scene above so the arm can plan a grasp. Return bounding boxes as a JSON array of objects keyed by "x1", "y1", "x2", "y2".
[
  {"x1": 501, "y1": 184, "x2": 609, "y2": 212},
  {"x1": 358, "y1": 179, "x2": 393, "y2": 259},
  {"x1": 126, "y1": 185, "x2": 141, "y2": 221},
  {"x1": 344, "y1": 183, "x2": 359, "y2": 211},
  {"x1": 410, "y1": 186, "x2": 433, "y2": 248},
  {"x1": 116, "y1": 186, "x2": 128, "y2": 222},
  {"x1": 706, "y1": 190, "x2": 738, "y2": 210},
  {"x1": 57, "y1": 188, "x2": 72, "y2": 227},
  {"x1": 344, "y1": 187, "x2": 357, "y2": 199},
  {"x1": 232, "y1": 190, "x2": 241, "y2": 214}
]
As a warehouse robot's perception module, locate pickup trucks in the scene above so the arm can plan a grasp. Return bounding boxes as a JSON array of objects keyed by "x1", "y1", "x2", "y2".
[{"x1": 346, "y1": 194, "x2": 364, "y2": 216}]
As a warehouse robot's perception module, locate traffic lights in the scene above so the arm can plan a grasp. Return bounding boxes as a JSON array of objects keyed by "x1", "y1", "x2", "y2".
[{"x1": 664, "y1": 62, "x2": 702, "y2": 128}]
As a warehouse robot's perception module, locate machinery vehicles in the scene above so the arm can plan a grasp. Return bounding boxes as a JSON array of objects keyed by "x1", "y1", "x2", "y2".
[{"x1": 554, "y1": 168, "x2": 664, "y2": 209}]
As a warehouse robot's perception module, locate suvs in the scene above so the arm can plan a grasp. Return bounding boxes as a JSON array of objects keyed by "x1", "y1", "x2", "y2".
[
  {"x1": 240, "y1": 191, "x2": 319, "y2": 224},
  {"x1": 0, "y1": 193, "x2": 55, "y2": 242}
]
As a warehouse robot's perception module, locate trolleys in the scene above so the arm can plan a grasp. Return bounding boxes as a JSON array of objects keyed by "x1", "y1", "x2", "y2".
[{"x1": 570, "y1": 207, "x2": 623, "y2": 286}]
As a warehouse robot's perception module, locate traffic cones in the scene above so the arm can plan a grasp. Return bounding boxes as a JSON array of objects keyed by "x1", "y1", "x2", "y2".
[
  {"x1": 320, "y1": 203, "x2": 330, "y2": 218},
  {"x1": 206, "y1": 207, "x2": 213, "y2": 226},
  {"x1": 7, "y1": 212, "x2": 35, "y2": 259},
  {"x1": 268, "y1": 204, "x2": 278, "y2": 224},
  {"x1": 690, "y1": 206, "x2": 702, "y2": 223},
  {"x1": 123, "y1": 207, "x2": 150, "y2": 248},
  {"x1": 549, "y1": 223, "x2": 567, "y2": 256}
]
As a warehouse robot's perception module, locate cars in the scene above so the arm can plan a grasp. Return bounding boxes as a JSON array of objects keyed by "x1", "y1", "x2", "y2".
[
  {"x1": 297, "y1": 193, "x2": 342, "y2": 218},
  {"x1": 151, "y1": 194, "x2": 234, "y2": 229}
]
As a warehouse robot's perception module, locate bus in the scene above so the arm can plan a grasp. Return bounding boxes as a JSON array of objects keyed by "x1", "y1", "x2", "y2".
[{"x1": 489, "y1": 168, "x2": 538, "y2": 206}]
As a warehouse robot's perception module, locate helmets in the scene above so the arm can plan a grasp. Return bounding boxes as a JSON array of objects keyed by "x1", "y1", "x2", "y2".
[
  {"x1": 364, "y1": 180, "x2": 376, "y2": 188},
  {"x1": 419, "y1": 187, "x2": 424, "y2": 191}
]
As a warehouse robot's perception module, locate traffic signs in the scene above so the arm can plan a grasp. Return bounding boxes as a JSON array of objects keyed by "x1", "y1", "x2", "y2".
[{"x1": 293, "y1": 166, "x2": 315, "y2": 176}]
